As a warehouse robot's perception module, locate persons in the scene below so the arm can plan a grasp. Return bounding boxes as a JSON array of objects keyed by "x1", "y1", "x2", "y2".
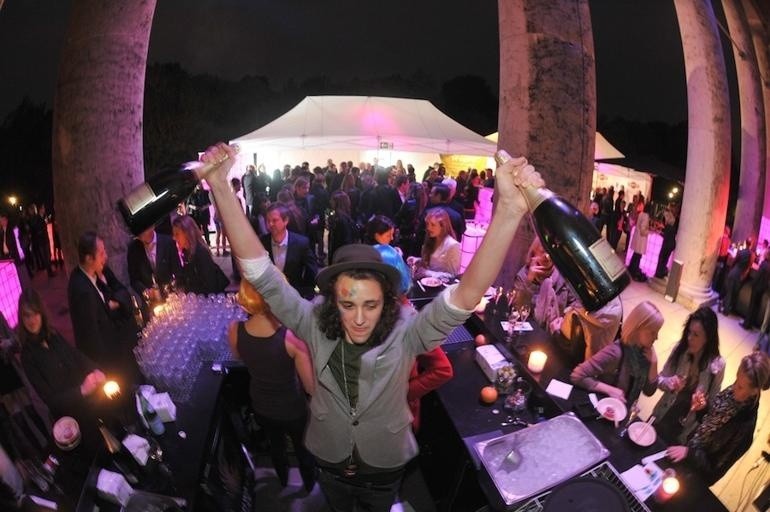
[{"x1": 0, "y1": 140, "x2": 770, "y2": 512}]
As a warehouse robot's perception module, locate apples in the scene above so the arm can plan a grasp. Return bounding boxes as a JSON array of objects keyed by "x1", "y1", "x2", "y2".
[
  {"x1": 480, "y1": 386, "x2": 498, "y2": 404},
  {"x1": 474, "y1": 334, "x2": 488, "y2": 346}
]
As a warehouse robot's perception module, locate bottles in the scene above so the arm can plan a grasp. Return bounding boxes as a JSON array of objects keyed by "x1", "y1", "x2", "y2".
[
  {"x1": 495, "y1": 149, "x2": 633, "y2": 310},
  {"x1": 134, "y1": 390, "x2": 163, "y2": 437},
  {"x1": 117, "y1": 146, "x2": 242, "y2": 237},
  {"x1": 93, "y1": 417, "x2": 150, "y2": 485}
]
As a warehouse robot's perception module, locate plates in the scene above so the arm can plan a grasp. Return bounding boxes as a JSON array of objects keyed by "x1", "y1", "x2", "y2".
[
  {"x1": 596, "y1": 396, "x2": 631, "y2": 421},
  {"x1": 628, "y1": 422, "x2": 657, "y2": 448},
  {"x1": 421, "y1": 274, "x2": 495, "y2": 299}
]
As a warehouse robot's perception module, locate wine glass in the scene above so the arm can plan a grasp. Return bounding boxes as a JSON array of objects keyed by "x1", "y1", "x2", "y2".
[{"x1": 500, "y1": 303, "x2": 533, "y2": 345}]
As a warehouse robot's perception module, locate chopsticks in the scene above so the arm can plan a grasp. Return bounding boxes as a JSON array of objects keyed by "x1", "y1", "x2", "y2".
[{"x1": 635, "y1": 416, "x2": 656, "y2": 443}]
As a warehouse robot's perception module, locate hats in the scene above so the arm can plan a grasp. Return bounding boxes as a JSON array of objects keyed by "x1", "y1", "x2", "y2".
[{"x1": 314, "y1": 243, "x2": 404, "y2": 286}]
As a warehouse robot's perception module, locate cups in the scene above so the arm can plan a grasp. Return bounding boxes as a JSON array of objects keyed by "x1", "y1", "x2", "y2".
[
  {"x1": 53, "y1": 417, "x2": 82, "y2": 451},
  {"x1": 498, "y1": 369, "x2": 531, "y2": 412},
  {"x1": 131, "y1": 284, "x2": 246, "y2": 390},
  {"x1": 143, "y1": 437, "x2": 162, "y2": 463}
]
